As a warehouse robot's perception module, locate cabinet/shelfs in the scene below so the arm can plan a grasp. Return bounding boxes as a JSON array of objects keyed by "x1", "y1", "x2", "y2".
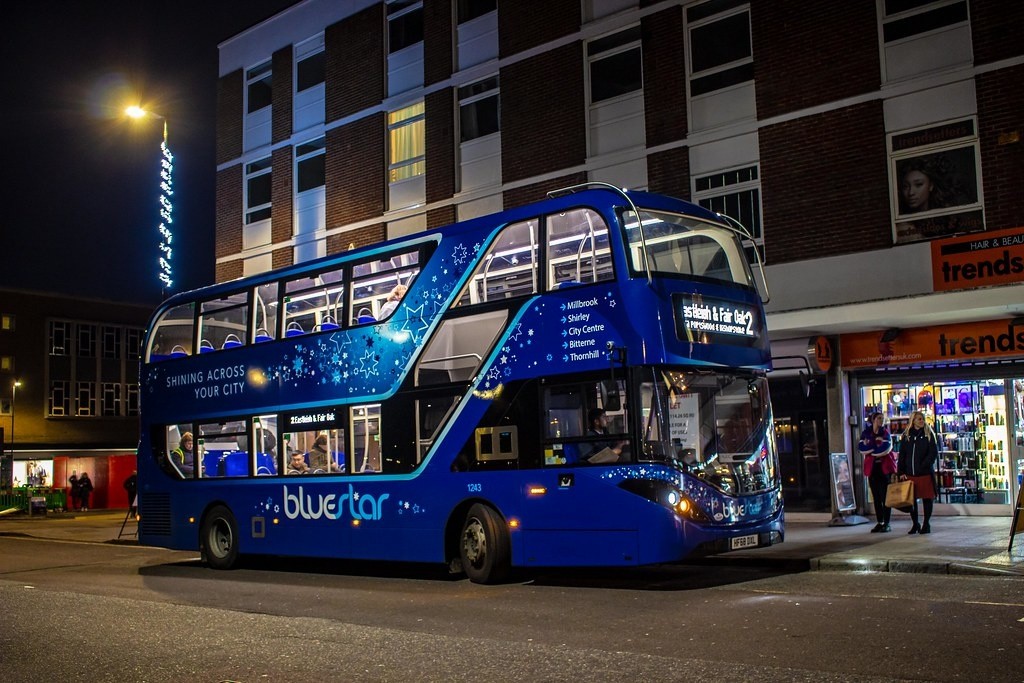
[{"x1": 870, "y1": 383, "x2": 986, "y2": 503}]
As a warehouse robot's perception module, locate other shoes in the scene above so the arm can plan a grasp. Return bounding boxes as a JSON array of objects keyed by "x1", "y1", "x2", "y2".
[
  {"x1": 871, "y1": 523, "x2": 884, "y2": 533},
  {"x1": 880, "y1": 525, "x2": 891, "y2": 532},
  {"x1": 81, "y1": 506, "x2": 87, "y2": 512}
]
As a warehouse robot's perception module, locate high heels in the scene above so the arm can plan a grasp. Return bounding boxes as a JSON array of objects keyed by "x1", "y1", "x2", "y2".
[
  {"x1": 907, "y1": 521, "x2": 921, "y2": 534},
  {"x1": 920, "y1": 522, "x2": 931, "y2": 535}
]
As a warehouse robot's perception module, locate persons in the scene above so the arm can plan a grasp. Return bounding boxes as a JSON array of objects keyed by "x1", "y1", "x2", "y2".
[
  {"x1": 378, "y1": 284, "x2": 408, "y2": 321},
  {"x1": 273, "y1": 444, "x2": 293, "y2": 471},
  {"x1": 287, "y1": 450, "x2": 310, "y2": 474},
  {"x1": 579, "y1": 408, "x2": 624, "y2": 456},
  {"x1": 858, "y1": 411, "x2": 897, "y2": 534},
  {"x1": 309, "y1": 435, "x2": 335, "y2": 471},
  {"x1": 897, "y1": 411, "x2": 938, "y2": 535},
  {"x1": 171, "y1": 432, "x2": 209, "y2": 478},
  {"x1": 123, "y1": 471, "x2": 137, "y2": 518},
  {"x1": 70, "y1": 472, "x2": 93, "y2": 511},
  {"x1": 903, "y1": 168, "x2": 960, "y2": 236}
]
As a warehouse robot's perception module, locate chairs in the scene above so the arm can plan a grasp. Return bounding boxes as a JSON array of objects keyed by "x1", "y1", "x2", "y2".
[
  {"x1": 146, "y1": 308, "x2": 376, "y2": 359},
  {"x1": 195, "y1": 447, "x2": 373, "y2": 474}
]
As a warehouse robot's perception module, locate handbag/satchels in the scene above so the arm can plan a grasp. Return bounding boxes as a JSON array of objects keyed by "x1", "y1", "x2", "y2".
[{"x1": 884, "y1": 479, "x2": 915, "y2": 512}]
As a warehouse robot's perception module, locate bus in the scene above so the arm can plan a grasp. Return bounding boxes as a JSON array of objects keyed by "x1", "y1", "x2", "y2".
[{"x1": 136, "y1": 181, "x2": 821, "y2": 583}]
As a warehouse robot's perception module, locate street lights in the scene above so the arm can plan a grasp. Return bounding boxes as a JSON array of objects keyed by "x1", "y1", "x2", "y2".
[
  {"x1": 11, "y1": 382, "x2": 24, "y2": 458},
  {"x1": 125, "y1": 106, "x2": 171, "y2": 297}
]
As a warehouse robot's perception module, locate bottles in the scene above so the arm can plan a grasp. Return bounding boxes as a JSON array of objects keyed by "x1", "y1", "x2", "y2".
[
  {"x1": 988, "y1": 440, "x2": 1003, "y2": 450},
  {"x1": 925, "y1": 415, "x2": 940, "y2": 434},
  {"x1": 934, "y1": 488, "x2": 983, "y2": 504},
  {"x1": 974, "y1": 417, "x2": 986, "y2": 451},
  {"x1": 989, "y1": 465, "x2": 1005, "y2": 476},
  {"x1": 886, "y1": 392, "x2": 916, "y2": 416},
  {"x1": 935, "y1": 471, "x2": 975, "y2": 486},
  {"x1": 936, "y1": 403, "x2": 972, "y2": 413},
  {"x1": 989, "y1": 478, "x2": 1009, "y2": 490},
  {"x1": 976, "y1": 412, "x2": 1005, "y2": 426},
  {"x1": 933, "y1": 453, "x2": 975, "y2": 471},
  {"x1": 864, "y1": 403, "x2": 882, "y2": 418},
  {"x1": 976, "y1": 451, "x2": 986, "y2": 469},
  {"x1": 955, "y1": 434, "x2": 974, "y2": 451},
  {"x1": 984, "y1": 382, "x2": 1004, "y2": 395},
  {"x1": 866, "y1": 420, "x2": 909, "y2": 434},
  {"x1": 990, "y1": 452, "x2": 1003, "y2": 463},
  {"x1": 892, "y1": 437, "x2": 901, "y2": 452},
  {"x1": 919, "y1": 392, "x2": 933, "y2": 412},
  {"x1": 941, "y1": 414, "x2": 973, "y2": 433},
  {"x1": 978, "y1": 471, "x2": 988, "y2": 490},
  {"x1": 937, "y1": 434, "x2": 957, "y2": 451}
]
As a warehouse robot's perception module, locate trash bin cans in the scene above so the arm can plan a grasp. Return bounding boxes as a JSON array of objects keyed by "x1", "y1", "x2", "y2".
[{"x1": 13, "y1": 484, "x2": 68, "y2": 513}]
